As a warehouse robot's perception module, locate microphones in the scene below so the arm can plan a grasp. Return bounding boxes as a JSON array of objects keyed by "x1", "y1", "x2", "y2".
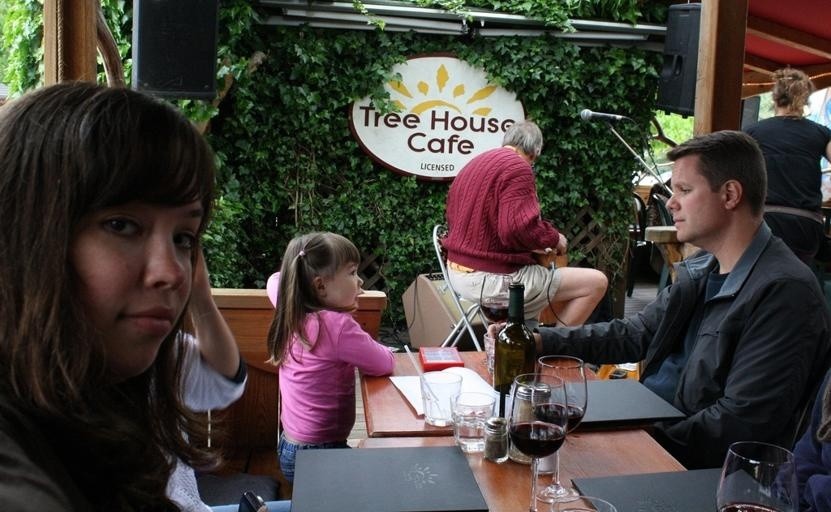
[{"x1": 581, "y1": 108, "x2": 636, "y2": 125}]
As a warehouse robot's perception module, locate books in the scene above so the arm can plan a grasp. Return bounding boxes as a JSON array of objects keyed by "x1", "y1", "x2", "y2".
[
  {"x1": 289, "y1": 443, "x2": 489, "y2": 511},
  {"x1": 537, "y1": 379, "x2": 688, "y2": 423},
  {"x1": 569, "y1": 463, "x2": 797, "y2": 512}
]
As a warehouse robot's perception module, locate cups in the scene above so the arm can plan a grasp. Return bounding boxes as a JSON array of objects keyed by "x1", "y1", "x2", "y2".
[
  {"x1": 715, "y1": 441, "x2": 798, "y2": 512},
  {"x1": 418, "y1": 369, "x2": 496, "y2": 455},
  {"x1": 484, "y1": 334, "x2": 496, "y2": 373},
  {"x1": 550, "y1": 494, "x2": 615, "y2": 512}
]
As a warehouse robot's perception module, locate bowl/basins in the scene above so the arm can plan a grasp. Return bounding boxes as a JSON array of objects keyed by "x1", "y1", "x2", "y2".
[{"x1": 481, "y1": 379, "x2": 552, "y2": 467}]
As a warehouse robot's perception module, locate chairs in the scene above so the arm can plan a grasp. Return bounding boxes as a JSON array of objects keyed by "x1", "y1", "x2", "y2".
[{"x1": 424, "y1": 223, "x2": 490, "y2": 354}]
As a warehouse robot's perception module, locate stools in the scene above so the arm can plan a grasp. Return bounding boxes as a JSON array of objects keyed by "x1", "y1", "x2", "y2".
[{"x1": 763, "y1": 204, "x2": 826, "y2": 264}]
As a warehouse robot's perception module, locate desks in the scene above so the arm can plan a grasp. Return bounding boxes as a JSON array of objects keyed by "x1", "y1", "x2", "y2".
[{"x1": 348, "y1": 341, "x2": 693, "y2": 510}]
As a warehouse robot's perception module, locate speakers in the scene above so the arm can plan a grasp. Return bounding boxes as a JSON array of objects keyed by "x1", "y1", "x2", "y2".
[
  {"x1": 130, "y1": 1, "x2": 219, "y2": 101},
  {"x1": 655, "y1": 2, "x2": 701, "y2": 119}
]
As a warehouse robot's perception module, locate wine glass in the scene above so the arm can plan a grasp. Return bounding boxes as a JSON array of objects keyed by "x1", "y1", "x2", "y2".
[
  {"x1": 481, "y1": 274, "x2": 513, "y2": 336},
  {"x1": 505, "y1": 354, "x2": 588, "y2": 512}
]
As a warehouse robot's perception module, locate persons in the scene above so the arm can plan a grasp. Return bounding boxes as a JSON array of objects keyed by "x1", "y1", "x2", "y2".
[
  {"x1": 444, "y1": 119, "x2": 609, "y2": 327},
  {"x1": 741, "y1": 67, "x2": 831, "y2": 272},
  {"x1": 486, "y1": 129, "x2": 830, "y2": 464},
  {"x1": 0, "y1": 80, "x2": 218, "y2": 511},
  {"x1": 769, "y1": 367, "x2": 830, "y2": 512},
  {"x1": 148, "y1": 245, "x2": 249, "y2": 509},
  {"x1": 263, "y1": 231, "x2": 397, "y2": 491}
]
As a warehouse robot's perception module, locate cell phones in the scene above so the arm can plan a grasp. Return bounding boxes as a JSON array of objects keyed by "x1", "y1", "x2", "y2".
[{"x1": 609, "y1": 368, "x2": 627, "y2": 379}]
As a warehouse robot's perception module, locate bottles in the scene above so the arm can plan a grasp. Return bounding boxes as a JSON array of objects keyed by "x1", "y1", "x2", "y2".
[{"x1": 494, "y1": 281, "x2": 537, "y2": 414}]
